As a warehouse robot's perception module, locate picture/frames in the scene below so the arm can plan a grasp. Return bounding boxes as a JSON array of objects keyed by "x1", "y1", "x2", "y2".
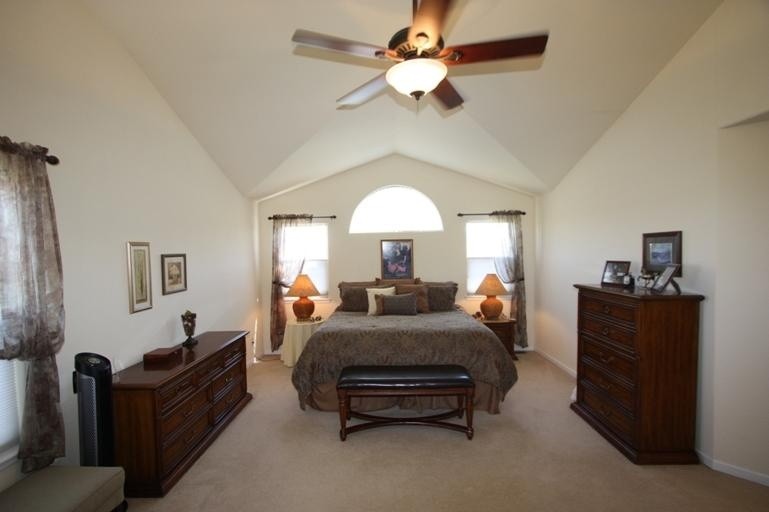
[
  {"x1": 381, "y1": 239, "x2": 414, "y2": 280},
  {"x1": 650, "y1": 263, "x2": 680, "y2": 292},
  {"x1": 642, "y1": 231, "x2": 683, "y2": 276},
  {"x1": 601, "y1": 261, "x2": 631, "y2": 286},
  {"x1": 161, "y1": 254, "x2": 188, "y2": 296},
  {"x1": 127, "y1": 241, "x2": 153, "y2": 314}
]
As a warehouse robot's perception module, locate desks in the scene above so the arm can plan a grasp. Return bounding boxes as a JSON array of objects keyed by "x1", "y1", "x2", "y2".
[{"x1": 280, "y1": 320, "x2": 324, "y2": 366}]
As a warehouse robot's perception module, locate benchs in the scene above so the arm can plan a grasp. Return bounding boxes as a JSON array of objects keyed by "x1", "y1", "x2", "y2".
[{"x1": 336, "y1": 365, "x2": 476, "y2": 441}]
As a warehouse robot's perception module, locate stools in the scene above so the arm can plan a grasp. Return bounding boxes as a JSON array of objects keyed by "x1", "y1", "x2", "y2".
[{"x1": 0, "y1": 465, "x2": 127, "y2": 512}]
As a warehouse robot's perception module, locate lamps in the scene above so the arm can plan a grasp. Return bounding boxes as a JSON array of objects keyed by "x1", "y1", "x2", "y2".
[
  {"x1": 475, "y1": 273, "x2": 509, "y2": 319},
  {"x1": 386, "y1": 57, "x2": 449, "y2": 102},
  {"x1": 285, "y1": 275, "x2": 322, "y2": 320},
  {"x1": 182, "y1": 312, "x2": 198, "y2": 347}
]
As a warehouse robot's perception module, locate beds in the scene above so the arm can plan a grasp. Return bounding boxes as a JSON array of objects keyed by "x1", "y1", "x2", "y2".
[{"x1": 291, "y1": 309, "x2": 518, "y2": 414}]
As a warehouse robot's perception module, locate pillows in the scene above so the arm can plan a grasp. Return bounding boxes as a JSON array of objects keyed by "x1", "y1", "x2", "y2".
[{"x1": 338, "y1": 281, "x2": 459, "y2": 315}]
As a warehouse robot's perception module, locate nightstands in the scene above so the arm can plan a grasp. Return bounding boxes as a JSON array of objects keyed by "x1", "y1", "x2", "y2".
[{"x1": 483, "y1": 318, "x2": 518, "y2": 361}]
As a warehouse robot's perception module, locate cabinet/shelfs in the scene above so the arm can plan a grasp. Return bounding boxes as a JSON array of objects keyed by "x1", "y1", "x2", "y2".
[
  {"x1": 570, "y1": 284, "x2": 704, "y2": 465},
  {"x1": 112, "y1": 332, "x2": 254, "y2": 497}
]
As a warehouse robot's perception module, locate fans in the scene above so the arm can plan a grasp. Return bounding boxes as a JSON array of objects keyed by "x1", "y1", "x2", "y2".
[{"x1": 291, "y1": 0, "x2": 549, "y2": 109}]
